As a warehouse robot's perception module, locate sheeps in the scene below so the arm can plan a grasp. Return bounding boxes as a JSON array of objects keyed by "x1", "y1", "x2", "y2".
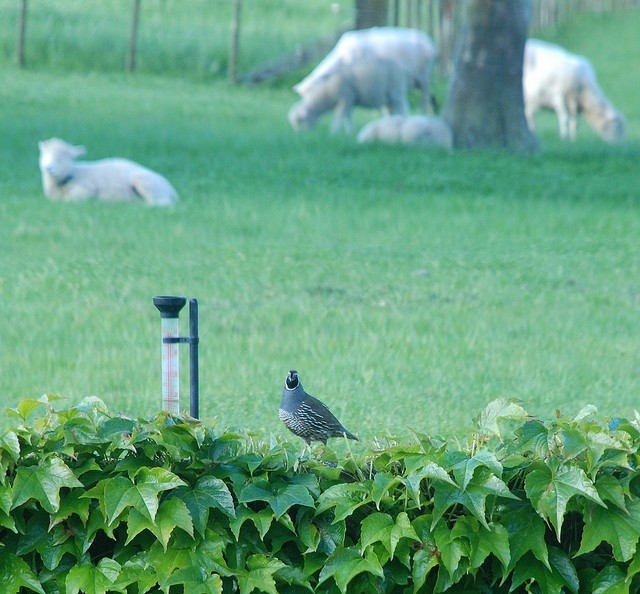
[
  {"x1": 288, "y1": 58, "x2": 416, "y2": 136},
  {"x1": 521, "y1": 38, "x2": 625, "y2": 142},
  {"x1": 38, "y1": 137, "x2": 180, "y2": 206},
  {"x1": 357, "y1": 114, "x2": 453, "y2": 152},
  {"x1": 292, "y1": 27, "x2": 441, "y2": 117}
]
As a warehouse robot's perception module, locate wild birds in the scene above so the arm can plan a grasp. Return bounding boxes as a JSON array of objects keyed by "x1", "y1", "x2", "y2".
[{"x1": 278, "y1": 370, "x2": 359, "y2": 461}]
